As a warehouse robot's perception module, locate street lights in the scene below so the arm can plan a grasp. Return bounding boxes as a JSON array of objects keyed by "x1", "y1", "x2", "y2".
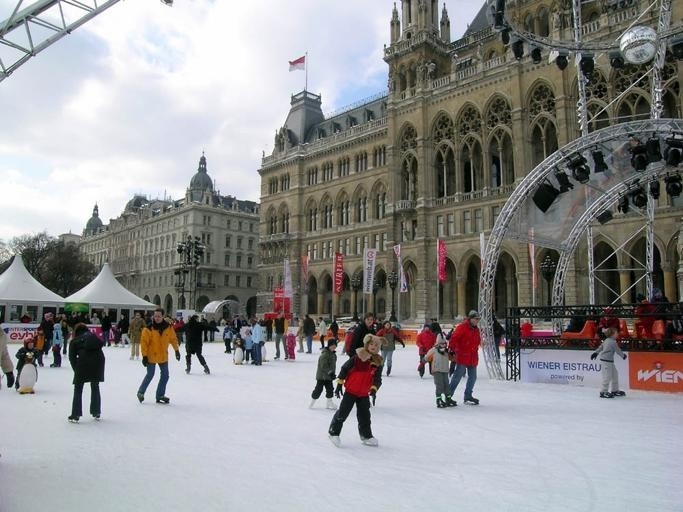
[
  {"x1": 371, "y1": 274, "x2": 384, "y2": 322},
  {"x1": 350, "y1": 271, "x2": 362, "y2": 321},
  {"x1": 385, "y1": 269, "x2": 398, "y2": 322},
  {"x1": 178, "y1": 235, "x2": 206, "y2": 312}
]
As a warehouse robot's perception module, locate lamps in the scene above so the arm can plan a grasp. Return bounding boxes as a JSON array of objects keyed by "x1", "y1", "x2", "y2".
[
  {"x1": 488, "y1": 1, "x2": 624, "y2": 73},
  {"x1": 533, "y1": 131, "x2": 683, "y2": 225}
]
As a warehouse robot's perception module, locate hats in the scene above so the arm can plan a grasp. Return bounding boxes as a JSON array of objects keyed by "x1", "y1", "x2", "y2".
[
  {"x1": 383, "y1": 321, "x2": 391, "y2": 328},
  {"x1": 44, "y1": 312, "x2": 53, "y2": 320},
  {"x1": 424, "y1": 323, "x2": 431, "y2": 328},
  {"x1": 653, "y1": 288, "x2": 663, "y2": 299},
  {"x1": 468, "y1": 310, "x2": 480, "y2": 319},
  {"x1": 436, "y1": 333, "x2": 446, "y2": 344},
  {"x1": 603, "y1": 308, "x2": 611, "y2": 314},
  {"x1": 328, "y1": 338, "x2": 337, "y2": 347},
  {"x1": 37, "y1": 327, "x2": 43, "y2": 331},
  {"x1": 637, "y1": 293, "x2": 646, "y2": 302},
  {"x1": 603, "y1": 327, "x2": 616, "y2": 338}
]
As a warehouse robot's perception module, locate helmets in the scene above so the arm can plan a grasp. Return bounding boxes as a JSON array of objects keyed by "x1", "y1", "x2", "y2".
[
  {"x1": 24, "y1": 337, "x2": 35, "y2": 348},
  {"x1": 53, "y1": 323, "x2": 61, "y2": 330}
]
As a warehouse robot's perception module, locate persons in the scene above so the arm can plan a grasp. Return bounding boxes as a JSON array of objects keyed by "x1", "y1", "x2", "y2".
[
  {"x1": 635, "y1": 293, "x2": 657, "y2": 326},
  {"x1": 415, "y1": 316, "x2": 465, "y2": 378},
  {"x1": 2, "y1": 305, "x2": 407, "y2": 422},
  {"x1": 589, "y1": 327, "x2": 627, "y2": 398},
  {"x1": 422, "y1": 333, "x2": 458, "y2": 407},
  {"x1": 651, "y1": 288, "x2": 667, "y2": 304},
  {"x1": 326, "y1": 334, "x2": 389, "y2": 449},
  {"x1": 447, "y1": 309, "x2": 481, "y2": 403},
  {"x1": 596, "y1": 307, "x2": 620, "y2": 338}
]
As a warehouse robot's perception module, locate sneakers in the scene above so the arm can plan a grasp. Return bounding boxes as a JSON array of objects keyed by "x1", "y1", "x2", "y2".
[
  {"x1": 445, "y1": 397, "x2": 457, "y2": 404},
  {"x1": 285, "y1": 357, "x2": 288, "y2": 359},
  {"x1": 361, "y1": 437, "x2": 378, "y2": 447},
  {"x1": 274, "y1": 357, "x2": 280, "y2": 359},
  {"x1": 600, "y1": 392, "x2": 614, "y2": 398},
  {"x1": 329, "y1": 436, "x2": 342, "y2": 448},
  {"x1": 611, "y1": 390, "x2": 626, "y2": 397},
  {"x1": 69, "y1": 415, "x2": 79, "y2": 420},
  {"x1": 437, "y1": 398, "x2": 446, "y2": 406},
  {"x1": 93, "y1": 414, "x2": 100, "y2": 418},
  {"x1": 50, "y1": 363, "x2": 60, "y2": 367},
  {"x1": 386, "y1": 366, "x2": 391, "y2": 374},
  {"x1": 137, "y1": 391, "x2": 144, "y2": 403},
  {"x1": 464, "y1": 396, "x2": 479, "y2": 404},
  {"x1": 420, "y1": 368, "x2": 424, "y2": 377},
  {"x1": 155, "y1": 395, "x2": 169, "y2": 401}
]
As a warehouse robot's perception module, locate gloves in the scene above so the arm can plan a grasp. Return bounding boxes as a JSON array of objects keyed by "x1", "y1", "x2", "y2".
[
  {"x1": 142, "y1": 356, "x2": 148, "y2": 367},
  {"x1": 175, "y1": 350, "x2": 180, "y2": 361},
  {"x1": 330, "y1": 372, "x2": 336, "y2": 380},
  {"x1": 591, "y1": 353, "x2": 599, "y2": 361},
  {"x1": 450, "y1": 368, "x2": 454, "y2": 377},
  {"x1": 334, "y1": 383, "x2": 343, "y2": 399},
  {"x1": 370, "y1": 388, "x2": 376, "y2": 406},
  {"x1": 623, "y1": 353, "x2": 627, "y2": 360},
  {"x1": 418, "y1": 361, "x2": 425, "y2": 370}
]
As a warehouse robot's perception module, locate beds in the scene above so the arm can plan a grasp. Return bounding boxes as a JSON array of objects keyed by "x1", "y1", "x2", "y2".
[{"x1": 558, "y1": 319, "x2": 666, "y2": 349}]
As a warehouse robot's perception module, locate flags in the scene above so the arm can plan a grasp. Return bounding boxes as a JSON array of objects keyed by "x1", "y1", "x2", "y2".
[{"x1": 288, "y1": 56, "x2": 305, "y2": 73}]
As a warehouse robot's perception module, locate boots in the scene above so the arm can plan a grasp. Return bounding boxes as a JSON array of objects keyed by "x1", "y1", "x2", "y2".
[
  {"x1": 309, "y1": 396, "x2": 316, "y2": 407},
  {"x1": 185, "y1": 364, "x2": 191, "y2": 371},
  {"x1": 326, "y1": 397, "x2": 338, "y2": 408},
  {"x1": 5, "y1": 371, "x2": 14, "y2": 388},
  {"x1": 204, "y1": 365, "x2": 210, "y2": 374}
]
